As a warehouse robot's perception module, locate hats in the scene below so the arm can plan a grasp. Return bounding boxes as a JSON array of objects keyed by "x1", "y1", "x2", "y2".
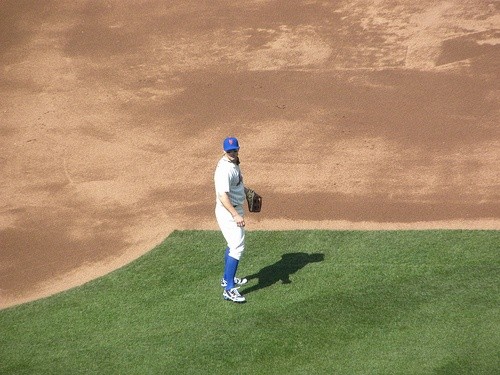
[{"x1": 223, "y1": 138, "x2": 240, "y2": 151}]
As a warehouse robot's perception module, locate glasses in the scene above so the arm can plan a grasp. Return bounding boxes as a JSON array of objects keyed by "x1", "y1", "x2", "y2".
[{"x1": 226, "y1": 149, "x2": 238, "y2": 154}]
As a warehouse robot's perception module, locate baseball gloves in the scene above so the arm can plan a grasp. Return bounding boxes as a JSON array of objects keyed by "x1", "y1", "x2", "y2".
[{"x1": 245, "y1": 187, "x2": 262, "y2": 213}]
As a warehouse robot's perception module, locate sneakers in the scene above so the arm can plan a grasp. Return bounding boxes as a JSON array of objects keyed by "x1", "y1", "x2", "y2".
[
  {"x1": 223, "y1": 288, "x2": 245, "y2": 303},
  {"x1": 221, "y1": 276, "x2": 248, "y2": 287}
]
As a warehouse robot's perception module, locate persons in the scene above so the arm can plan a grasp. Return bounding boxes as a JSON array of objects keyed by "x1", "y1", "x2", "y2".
[{"x1": 214, "y1": 137, "x2": 251, "y2": 304}]
